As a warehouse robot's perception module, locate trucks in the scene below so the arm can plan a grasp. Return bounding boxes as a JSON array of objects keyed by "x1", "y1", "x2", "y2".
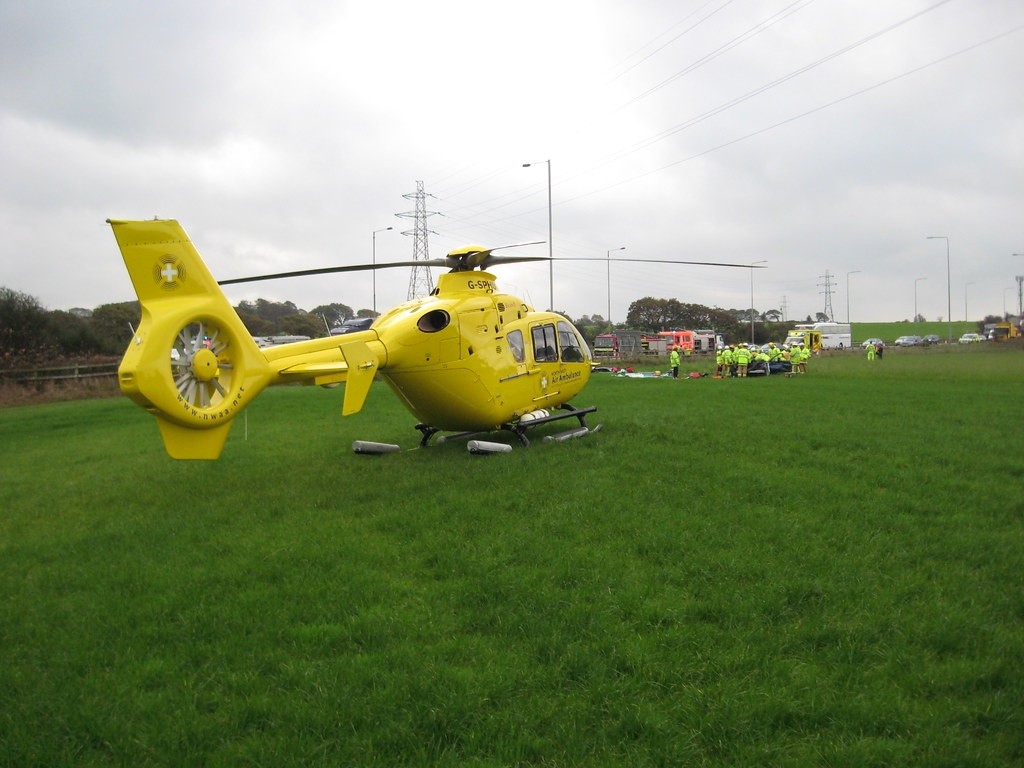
[{"x1": 189, "y1": 332, "x2": 311, "y2": 352}]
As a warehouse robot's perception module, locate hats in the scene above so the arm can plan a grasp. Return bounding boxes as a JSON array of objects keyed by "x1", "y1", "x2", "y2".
[{"x1": 783, "y1": 344, "x2": 787, "y2": 349}]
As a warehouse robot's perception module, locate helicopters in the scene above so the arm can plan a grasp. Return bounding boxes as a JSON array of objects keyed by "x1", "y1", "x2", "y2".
[{"x1": 100, "y1": 219, "x2": 769, "y2": 460}]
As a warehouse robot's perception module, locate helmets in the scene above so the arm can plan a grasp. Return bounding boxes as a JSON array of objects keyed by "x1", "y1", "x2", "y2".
[
  {"x1": 718, "y1": 346, "x2": 722, "y2": 350},
  {"x1": 799, "y1": 342, "x2": 805, "y2": 346},
  {"x1": 730, "y1": 344, "x2": 735, "y2": 349},
  {"x1": 768, "y1": 342, "x2": 774, "y2": 348},
  {"x1": 673, "y1": 345, "x2": 678, "y2": 349},
  {"x1": 738, "y1": 343, "x2": 743, "y2": 349},
  {"x1": 725, "y1": 345, "x2": 729, "y2": 349},
  {"x1": 744, "y1": 343, "x2": 748, "y2": 346},
  {"x1": 792, "y1": 342, "x2": 798, "y2": 345},
  {"x1": 751, "y1": 347, "x2": 755, "y2": 351}
]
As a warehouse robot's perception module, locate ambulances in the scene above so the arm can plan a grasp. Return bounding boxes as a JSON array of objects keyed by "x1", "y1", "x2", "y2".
[{"x1": 782, "y1": 329, "x2": 822, "y2": 356}]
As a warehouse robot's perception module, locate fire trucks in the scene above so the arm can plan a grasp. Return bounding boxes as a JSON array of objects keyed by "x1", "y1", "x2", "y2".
[{"x1": 591, "y1": 331, "x2": 668, "y2": 359}]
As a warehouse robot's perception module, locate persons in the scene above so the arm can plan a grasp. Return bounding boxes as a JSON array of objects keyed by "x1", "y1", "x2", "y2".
[
  {"x1": 678, "y1": 345, "x2": 684, "y2": 361},
  {"x1": 865, "y1": 340, "x2": 883, "y2": 360},
  {"x1": 716, "y1": 339, "x2": 820, "y2": 379},
  {"x1": 670, "y1": 347, "x2": 680, "y2": 380}
]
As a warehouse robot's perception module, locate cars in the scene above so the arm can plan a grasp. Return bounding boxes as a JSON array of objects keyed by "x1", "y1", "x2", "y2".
[
  {"x1": 762, "y1": 342, "x2": 784, "y2": 354},
  {"x1": 859, "y1": 321, "x2": 1022, "y2": 349},
  {"x1": 330, "y1": 318, "x2": 374, "y2": 335}
]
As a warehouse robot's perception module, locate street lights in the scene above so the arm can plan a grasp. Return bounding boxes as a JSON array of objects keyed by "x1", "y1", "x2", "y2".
[
  {"x1": 927, "y1": 236, "x2": 952, "y2": 345},
  {"x1": 846, "y1": 270, "x2": 862, "y2": 323},
  {"x1": 522, "y1": 159, "x2": 555, "y2": 312},
  {"x1": 373, "y1": 226, "x2": 393, "y2": 321},
  {"x1": 606, "y1": 247, "x2": 627, "y2": 334},
  {"x1": 750, "y1": 259, "x2": 768, "y2": 345}
]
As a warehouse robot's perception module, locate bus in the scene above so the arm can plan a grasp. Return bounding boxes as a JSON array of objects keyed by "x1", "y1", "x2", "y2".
[
  {"x1": 658, "y1": 329, "x2": 694, "y2": 355},
  {"x1": 794, "y1": 322, "x2": 851, "y2": 350}
]
